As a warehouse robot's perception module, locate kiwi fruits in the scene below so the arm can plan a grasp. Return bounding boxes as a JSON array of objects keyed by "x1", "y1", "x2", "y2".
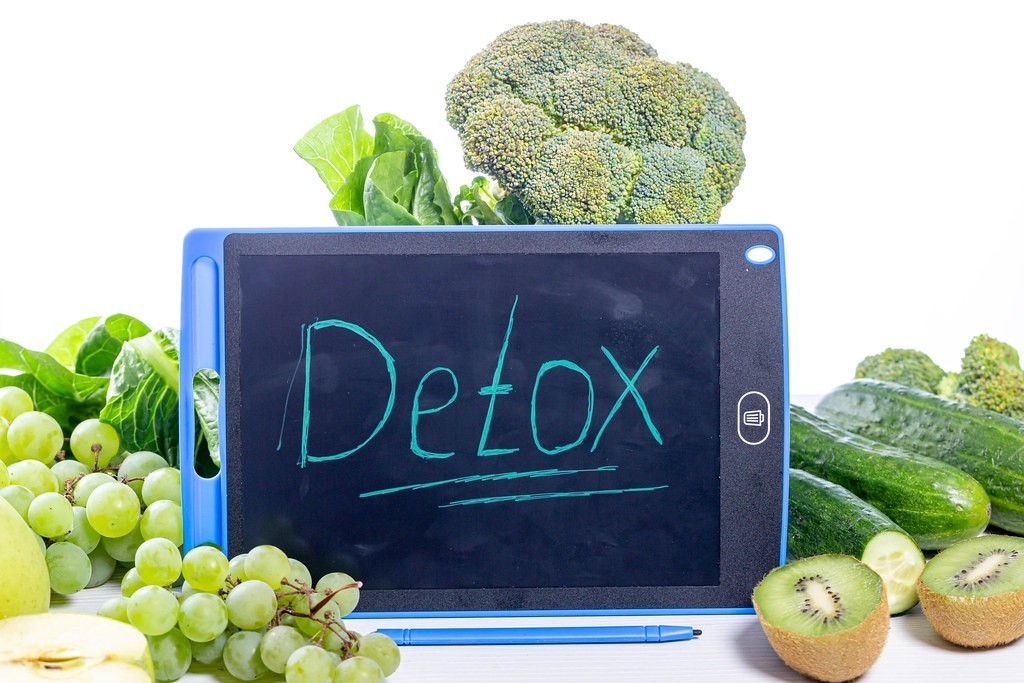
[
  {"x1": 751, "y1": 553, "x2": 889, "y2": 683},
  {"x1": 916, "y1": 535, "x2": 1024, "y2": 648}
]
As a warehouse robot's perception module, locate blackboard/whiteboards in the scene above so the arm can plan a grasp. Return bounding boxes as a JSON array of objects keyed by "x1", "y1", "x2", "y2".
[{"x1": 177, "y1": 220, "x2": 793, "y2": 617}]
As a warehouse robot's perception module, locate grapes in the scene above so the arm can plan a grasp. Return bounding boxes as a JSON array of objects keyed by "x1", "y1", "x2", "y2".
[{"x1": 0, "y1": 384, "x2": 395, "y2": 683}]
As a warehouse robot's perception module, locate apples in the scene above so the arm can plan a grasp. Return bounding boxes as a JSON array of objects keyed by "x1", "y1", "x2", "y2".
[
  {"x1": 0, "y1": 612, "x2": 155, "y2": 682},
  {"x1": 0, "y1": 496, "x2": 51, "y2": 620}
]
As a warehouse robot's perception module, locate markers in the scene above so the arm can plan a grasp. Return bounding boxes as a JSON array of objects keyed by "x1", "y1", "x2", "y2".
[{"x1": 375, "y1": 625, "x2": 705, "y2": 650}]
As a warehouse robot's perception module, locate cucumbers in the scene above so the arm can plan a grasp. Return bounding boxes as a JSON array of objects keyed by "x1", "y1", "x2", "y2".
[{"x1": 787, "y1": 377, "x2": 1024, "y2": 614}]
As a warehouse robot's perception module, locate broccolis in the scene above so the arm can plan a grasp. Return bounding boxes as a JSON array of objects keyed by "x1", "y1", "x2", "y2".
[
  {"x1": 854, "y1": 334, "x2": 1024, "y2": 421},
  {"x1": 443, "y1": 18, "x2": 745, "y2": 226}
]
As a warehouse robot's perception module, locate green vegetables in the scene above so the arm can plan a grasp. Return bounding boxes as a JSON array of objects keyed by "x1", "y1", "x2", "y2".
[
  {"x1": 293, "y1": 104, "x2": 537, "y2": 225},
  {"x1": 0, "y1": 310, "x2": 223, "y2": 477}
]
